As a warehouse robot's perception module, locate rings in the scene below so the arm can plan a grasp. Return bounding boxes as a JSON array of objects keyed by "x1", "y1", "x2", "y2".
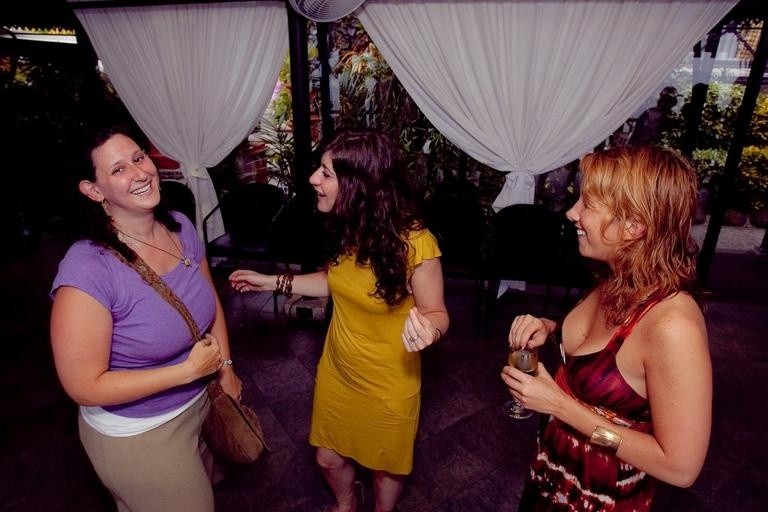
[{"x1": 408, "y1": 336, "x2": 418, "y2": 341}]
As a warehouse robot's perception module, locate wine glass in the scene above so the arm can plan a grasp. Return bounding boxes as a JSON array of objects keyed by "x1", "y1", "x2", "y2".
[{"x1": 501, "y1": 348, "x2": 539, "y2": 420}]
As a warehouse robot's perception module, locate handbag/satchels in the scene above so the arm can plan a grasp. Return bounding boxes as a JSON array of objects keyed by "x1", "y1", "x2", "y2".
[{"x1": 200, "y1": 373, "x2": 274, "y2": 468}]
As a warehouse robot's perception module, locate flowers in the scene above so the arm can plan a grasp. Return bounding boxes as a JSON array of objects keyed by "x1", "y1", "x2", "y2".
[{"x1": 692, "y1": 144, "x2": 768, "y2": 193}]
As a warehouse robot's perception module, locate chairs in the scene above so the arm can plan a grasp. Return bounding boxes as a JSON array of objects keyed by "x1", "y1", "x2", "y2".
[
  {"x1": 202, "y1": 171, "x2": 294, "y2": 317},
  {"x1": 433, "y1": 201, "x2": 569, "y2": 333}
]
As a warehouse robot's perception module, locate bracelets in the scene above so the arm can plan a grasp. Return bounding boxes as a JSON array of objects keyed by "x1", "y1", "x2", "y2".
[
  {"x1": 589, "y1": 416, "x2": 633, "y2": 457},
  {"x1": 435, "y1": 327, "x2": 443, "y2": 344},
  {"x1": 222, "y1": 358, "x2": 233, "y2": 367},
  {"x1": 274, "y1": 273, "x2": 294, "y2": 295}
]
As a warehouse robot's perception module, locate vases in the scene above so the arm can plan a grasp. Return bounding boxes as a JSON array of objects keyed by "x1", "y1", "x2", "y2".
[{"x1": 693, "y1": 193, "x2": 768, "y2": 228}]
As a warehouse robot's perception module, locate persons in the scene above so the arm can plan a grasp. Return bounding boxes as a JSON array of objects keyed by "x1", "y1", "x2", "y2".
[
  {"x1": 499, "y1": 142, "x2": 715, "y2": 511},
  {"x1": 227, "y1": 126, "x2": 453, "y2": 511},
  {"x1": 46, "y1": 122, "x2": 243, "y2": 512},
  {"x1": 677, "y1": 82, "x2": 709, "y2": 156},
  {"x1": 626, "y1": 86, "x2": 687, "y2": 149}
]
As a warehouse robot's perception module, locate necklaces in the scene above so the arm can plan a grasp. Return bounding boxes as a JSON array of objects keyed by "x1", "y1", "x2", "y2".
[{"x1": 110, "y1": 218, "x2": 193, "y2": 269}]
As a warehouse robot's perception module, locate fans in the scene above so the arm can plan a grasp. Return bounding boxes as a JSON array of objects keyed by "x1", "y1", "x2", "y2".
[{"x1": 289, "y1": 0, "x2": 367, "y2": 22}]
